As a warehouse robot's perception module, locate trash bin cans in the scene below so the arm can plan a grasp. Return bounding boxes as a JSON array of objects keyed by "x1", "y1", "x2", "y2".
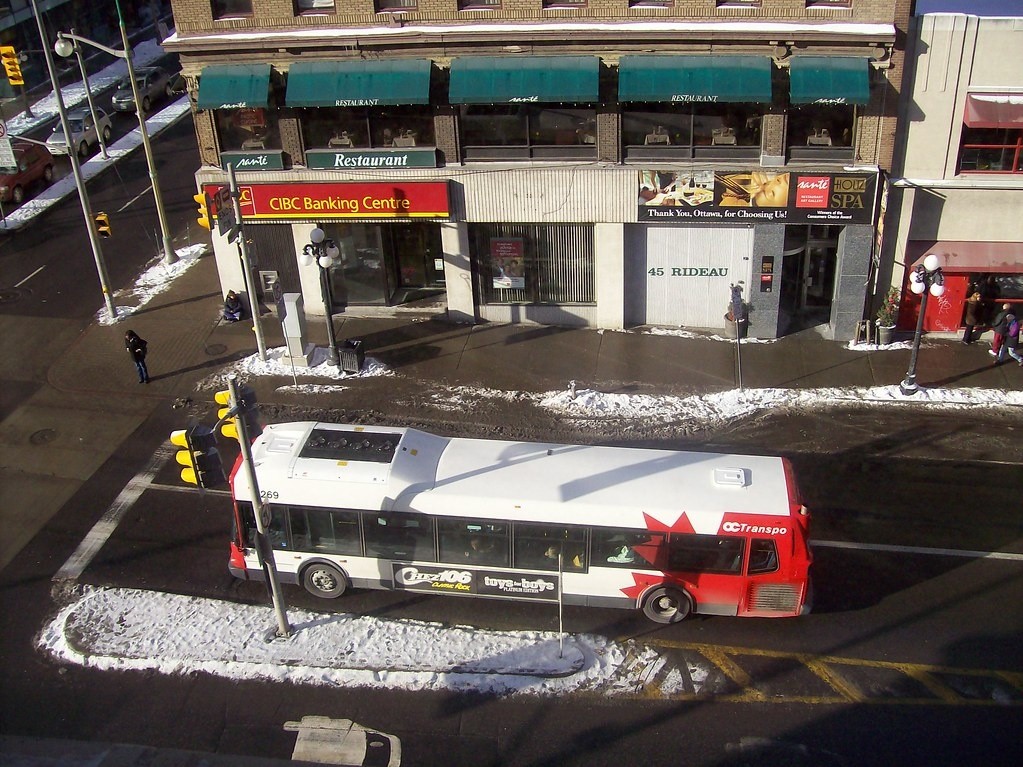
[{"x1": 339, "y1": 339, "x2": 365, "y2": 373}]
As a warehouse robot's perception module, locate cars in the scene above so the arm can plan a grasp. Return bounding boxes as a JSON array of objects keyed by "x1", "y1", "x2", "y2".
[
  {"x1": 45, "y1": 106, "x2": 113, "y2": 157},
  {"x1": 0, "y1": 142, "x2": 53, "y2": 205}
]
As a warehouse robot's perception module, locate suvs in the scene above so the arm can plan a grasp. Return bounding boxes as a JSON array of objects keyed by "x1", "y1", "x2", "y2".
[{"x1": 112, "y1": 66, "x2": 173, "y2": 113}]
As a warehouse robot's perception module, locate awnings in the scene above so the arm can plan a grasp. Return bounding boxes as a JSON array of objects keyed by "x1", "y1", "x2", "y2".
[
  {"x1": 196, "y1": 63, "x2": 271, "y2": 110},
  {"x1": 286, "y1": 57, "x2": 431, "y2": 108},
  {"x1": 963, "y1": 92, "x2": 1023, "y2": 129},
  {"x1": 618, "y1": 55, "x2": 774, "y2": 103},
  {"x1": 788, "y1": 55, "x2": 870, "y2": 105},
  {"x1": 448, "y1": 56, "x2": 600, "y2": 104}
]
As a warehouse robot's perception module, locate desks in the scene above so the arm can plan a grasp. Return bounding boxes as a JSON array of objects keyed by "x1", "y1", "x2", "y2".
[
  {"x1": 644, "y1": 133, "x2": 672, "y2": 146},
  {"x1": 241, "y1": 138, "x2": 269, "y2": 151},
  {"x1": 328, "y1": 136, "x2": 355, "y2": 149},
  {"x1": 711, "y1": 133, "x2": 738, "y2": 146},
  {"x1": 391, "y1": 135, "x2": 418, "y2": 148},
  {"x1": 581, "y1": 133, "x2": 596, "y2": 145},
  {"x1": 806, "y1": 134, "x2": 832, "y2": 147}
]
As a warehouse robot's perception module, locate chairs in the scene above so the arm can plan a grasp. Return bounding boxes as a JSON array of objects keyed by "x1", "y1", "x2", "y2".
[{"x1": 731, "y1": 552, "x2": 740, "y2": 569}]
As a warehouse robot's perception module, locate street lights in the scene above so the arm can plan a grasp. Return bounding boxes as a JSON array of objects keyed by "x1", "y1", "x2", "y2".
[
  {"x1": 898, "y1": 254, "x2": 945, "y2": 396},
  {"x1": 54, "y1": 30, "x2": 179, "y2": 264},
  {"x1": 301, "y1": 228, "x2": 342, "y2": 368}
]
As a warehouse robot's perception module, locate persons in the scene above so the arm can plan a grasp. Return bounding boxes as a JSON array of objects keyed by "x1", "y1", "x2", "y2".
[
  {"x1": 224, "y1": 291, "x2": 242, "y2": 321},
  {"x1": 752, "y1": 172, "x2": 790, "y2": 206},
  {"x1": 376, "y1": 521, "x2": 650, "y2": 570},
  {"x1": 493, "y1": 259, "x2": 523, "y2": 277},
  {"x1": 124, "y1": 329, "x2": 150, "y2": 383},
  {"x1": 651, "y1": 170, "x2": 677, "y2": 193},
  {"x1": 961, "y1": 293, "x2": 1023, "y2": 367}
]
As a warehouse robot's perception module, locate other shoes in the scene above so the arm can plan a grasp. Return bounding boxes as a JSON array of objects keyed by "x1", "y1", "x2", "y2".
[{"x1": 962, "y1": 340, "x2": 975, "y2": 346}]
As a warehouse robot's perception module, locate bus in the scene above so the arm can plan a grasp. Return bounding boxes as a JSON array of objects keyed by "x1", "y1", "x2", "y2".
[{"x1": 227, "y1": 422, "x2": 812, "y2": 625}]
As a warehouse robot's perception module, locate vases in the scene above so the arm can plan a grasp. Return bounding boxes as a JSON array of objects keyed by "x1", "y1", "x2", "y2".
[
  {"x1": 878, "y1": 324, "x2": 897, "y2": 345},
  {"x1": 723, "y1": 312, "x2": 747, "y2": 340}
]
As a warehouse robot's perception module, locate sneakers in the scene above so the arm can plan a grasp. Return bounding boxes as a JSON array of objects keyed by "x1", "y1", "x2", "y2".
[
  {"x1": 223, "y1": 315, "x2": 227, "y2": 320},
  {"x1": 994, "y1": 361, "x2": 1003, "y2": 365},
  {"x1": 139, "y1": 380, "x2": 144, "y2": 384},
  {"x1": 231, "y1": 319, "x2": 238, "y2": 323},
  {"x1": 989, "y1": 350, "x2": 997, "y2": 355},
  {"x1": 1019, "y1": 362, "x2": 1023, "y2": 366},
  {"x1": 144, "y1": 380, "x2": 149, "y2": 384}
]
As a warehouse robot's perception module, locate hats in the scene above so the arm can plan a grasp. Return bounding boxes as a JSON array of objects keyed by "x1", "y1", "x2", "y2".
[{"x1": 228, "y1": 290, "x2": 235, "y2": 296}]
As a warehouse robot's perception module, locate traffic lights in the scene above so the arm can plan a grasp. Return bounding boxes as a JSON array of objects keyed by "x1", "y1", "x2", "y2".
[
  {"x1": 171, "y1": 425, "x2": 201, "y2": 489},
  {"x1": 95, "y1": 213, "x2": 111, "y2": 236},
  {"x1": 0, "y1": 46, "x2": 24, "y2": 86},
  {"x1": 193, "y1": 190, "x2": 214, "y2": 232},
  {"x1": 214, "y1": 389, "x2": 239, "y2": 441}
]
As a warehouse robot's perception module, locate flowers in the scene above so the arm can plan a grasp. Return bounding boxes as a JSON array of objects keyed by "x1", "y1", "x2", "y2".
[
  {"x1": 727, "y1": 280, "x2": 752, "y2": 320},
  {"x1": 876, "y1": 285, "x2": 902, "y2": 327}
]
